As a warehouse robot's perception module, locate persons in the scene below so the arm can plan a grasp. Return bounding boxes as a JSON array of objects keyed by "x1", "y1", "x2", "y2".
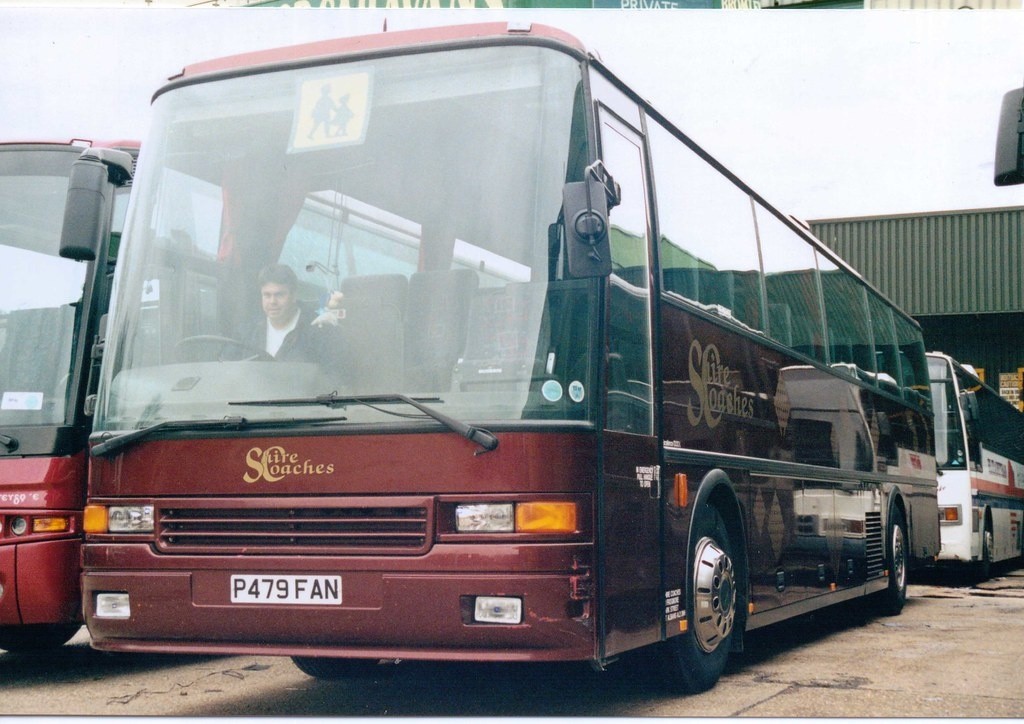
[{"x1": 218, "y1": 262, "x2": 338, "y2": 373}]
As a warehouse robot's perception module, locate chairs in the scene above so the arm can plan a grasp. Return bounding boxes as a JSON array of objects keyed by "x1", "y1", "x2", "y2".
[{"x1": 340, "y1": 267, "x2": 541, "y2": 393}]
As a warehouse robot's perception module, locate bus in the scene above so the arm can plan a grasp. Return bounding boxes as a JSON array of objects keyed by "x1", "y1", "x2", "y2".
[
  {"x1": 924, "y1": 349, "x2": 1024, "y2": 584},
  {"x1": 56, "y1": 19, "x2": 945, "y2": 699},
  {"x1": 0, "y1": 134, "x2": 528, "y2": 658}
]
